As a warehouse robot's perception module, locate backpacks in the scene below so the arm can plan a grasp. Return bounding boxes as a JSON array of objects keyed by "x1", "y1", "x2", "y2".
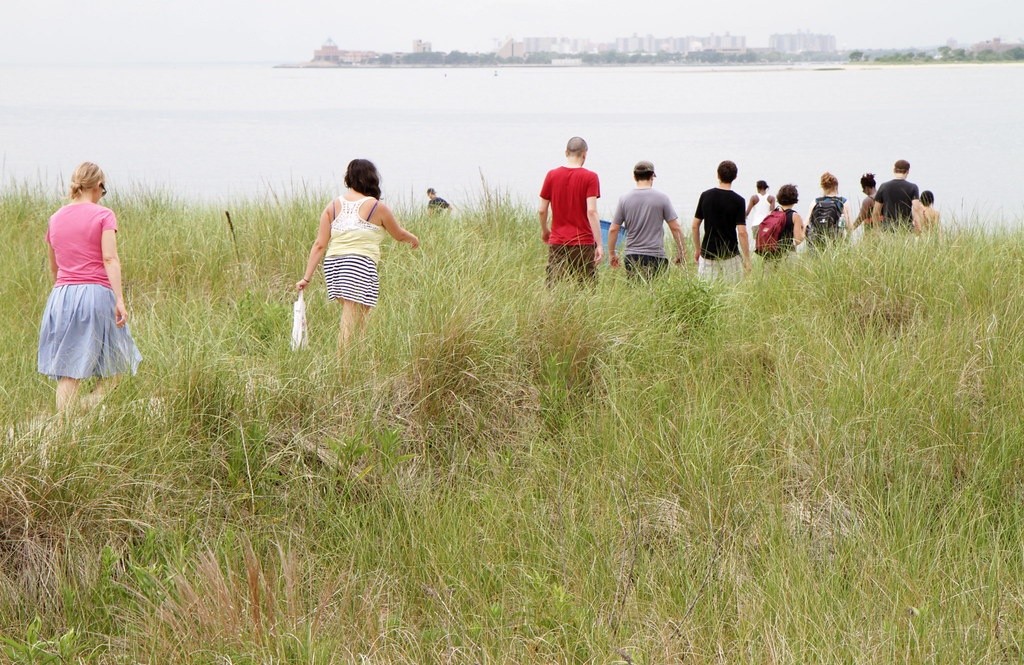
[
  {"x1": 809, "y1": 197, "x2": 844, "y2": 235},
  {"x1": 755, "y1": 205, "x2": 787, "y2": 257}
]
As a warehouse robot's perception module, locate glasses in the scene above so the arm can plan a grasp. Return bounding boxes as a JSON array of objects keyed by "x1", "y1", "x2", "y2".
[{"x1": 99, "y1": 183, "x2": 107, "y2": 196}]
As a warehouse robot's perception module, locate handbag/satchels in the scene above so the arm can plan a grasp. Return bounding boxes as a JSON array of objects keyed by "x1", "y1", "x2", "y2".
[{"x1": 292, "y1": 289, "x2": 308, "y2": 350}]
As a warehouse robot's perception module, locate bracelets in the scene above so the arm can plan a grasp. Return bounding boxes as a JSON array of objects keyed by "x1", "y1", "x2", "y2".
[{"x1": 304, "y1": 279, "x2": 309, "y2": 283}]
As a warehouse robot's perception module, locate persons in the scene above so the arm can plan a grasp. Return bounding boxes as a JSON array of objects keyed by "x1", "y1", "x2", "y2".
[
  {"x1": 427, "y1": 188, "x2": 452, "y2": 216},
  {"x1": 763, "y1": 184, "x2": 805, "y2": 273},
  {"x1": 852, "y1": 173, "x2": 877, "y2": 237},
  {"x1": 608, "y1": 161, "x2": 686, "y2": 287},
  {"x1": 809, "y1": 172, "x2": 851, "y2": 252},
  {"x1": 37, "y1": 162, "x2": 143, "y2": 413},
  {"x1": 745, "y1": 180, "x2": 775, "y2": 239},
  {"x1": 912, "y1": 190, "x2": 940, "y2": 232},
  {"x1": 295, "y1": 158, "x2": 419, "y2": 353},
  {"x1": 692, "y1": 160, "x2": 751, "y2": 284},
  {"x1": 872, "y1": 160, "x2": 922, "y2": 233},
  {"x1": 538, "y1": 136, "x2": 604, "y2": 295}
]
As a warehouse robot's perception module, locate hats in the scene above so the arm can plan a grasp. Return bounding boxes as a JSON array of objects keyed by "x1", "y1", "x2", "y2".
[
  {"x1": 634, "y1": 160, "x2": 656, "y2": 178},
  {"x1": 895, "y1": 160, "x2": 910, "y2": 170}
]
